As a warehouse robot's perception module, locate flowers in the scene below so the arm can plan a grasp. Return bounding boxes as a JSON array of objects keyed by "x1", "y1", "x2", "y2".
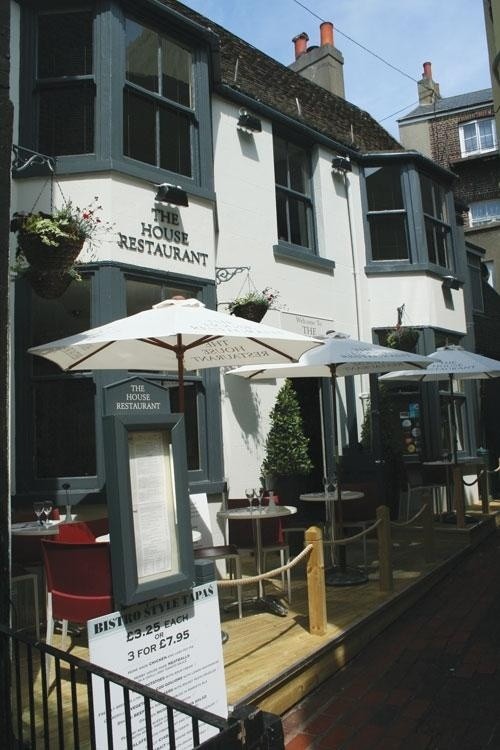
[
  {"x1": 14, "y1": 196, "x2": 117, "y2": 264},
  {"x1": 225, "y1": 267, "x2": 278, "y2": 315}
]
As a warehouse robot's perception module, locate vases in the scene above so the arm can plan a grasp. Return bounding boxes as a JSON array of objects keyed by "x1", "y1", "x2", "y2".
[
  {"x1": 233, "y1": 304, "x2": 268, "y2": 323},
  {"x1": 18, "y1": 234, "x2": 84, "y2": 266}
]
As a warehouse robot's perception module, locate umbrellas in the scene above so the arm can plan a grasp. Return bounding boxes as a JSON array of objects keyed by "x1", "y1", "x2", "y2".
[
  {"x1": 25, "y1": 292, "x2": 325, "y2": 413},
  {"x1": 224, "y1": 327, "x2": 444, "y2": 571},
  {"x1": 377, "y1": 344, "x2": 500, "y2": 460}
]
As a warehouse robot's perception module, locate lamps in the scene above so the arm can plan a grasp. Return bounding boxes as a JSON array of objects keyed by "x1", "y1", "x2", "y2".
[
  {"x1": 237, "y1": 106, "x2": 261, "y2": 133},
  {"x1": 332, "y1": 155, "x2": 352, "y2": 172},
  {"x1": 154, "y1": 182, "x2": 188, "y2": 207},
  {"x1": 443, "y1": 275, "x2": 464, "y2": 290}
]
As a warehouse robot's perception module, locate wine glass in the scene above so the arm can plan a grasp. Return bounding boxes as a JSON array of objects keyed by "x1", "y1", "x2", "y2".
[
  {"x1": 323, "y1": 476, "x2": 337, "y2": 497},
  {"x1": 245, "y1": 487, "x2": 265, "y2": 511},
  {"x1": 33, "y1": 501, "x2": 52, "y2": 527},
  {"x1": 441, "y1": 447, "x2": 449, "y2": 465}
]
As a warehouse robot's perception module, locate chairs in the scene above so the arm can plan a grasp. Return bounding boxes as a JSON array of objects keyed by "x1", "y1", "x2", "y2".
[
  {"x1": 40, "y1": 538, "x2": 113, "y2": 698},
  {"x1": 59, "y1": 517, "x2": 109, "y2": 543},
  {"x1": 195, "y1": 545, "x2": 243, "y2": 618},
  {"x1": 11, "y1": 565, "x2": 40, "y2": 639}
]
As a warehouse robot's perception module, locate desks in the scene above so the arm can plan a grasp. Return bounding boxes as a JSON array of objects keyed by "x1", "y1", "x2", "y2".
[
  {"x1": 12, "y1": 514, "x2": 77, "y2": 535},
  {"x1": 300, "y1": 491, "x2": 363, "y2": 568},
  {"x1": 217, "y1": 505, "x2": 297, "y2": 617},
  {"x1": 95, "y1": 530, "x2": 201, "y2": 543}
]
